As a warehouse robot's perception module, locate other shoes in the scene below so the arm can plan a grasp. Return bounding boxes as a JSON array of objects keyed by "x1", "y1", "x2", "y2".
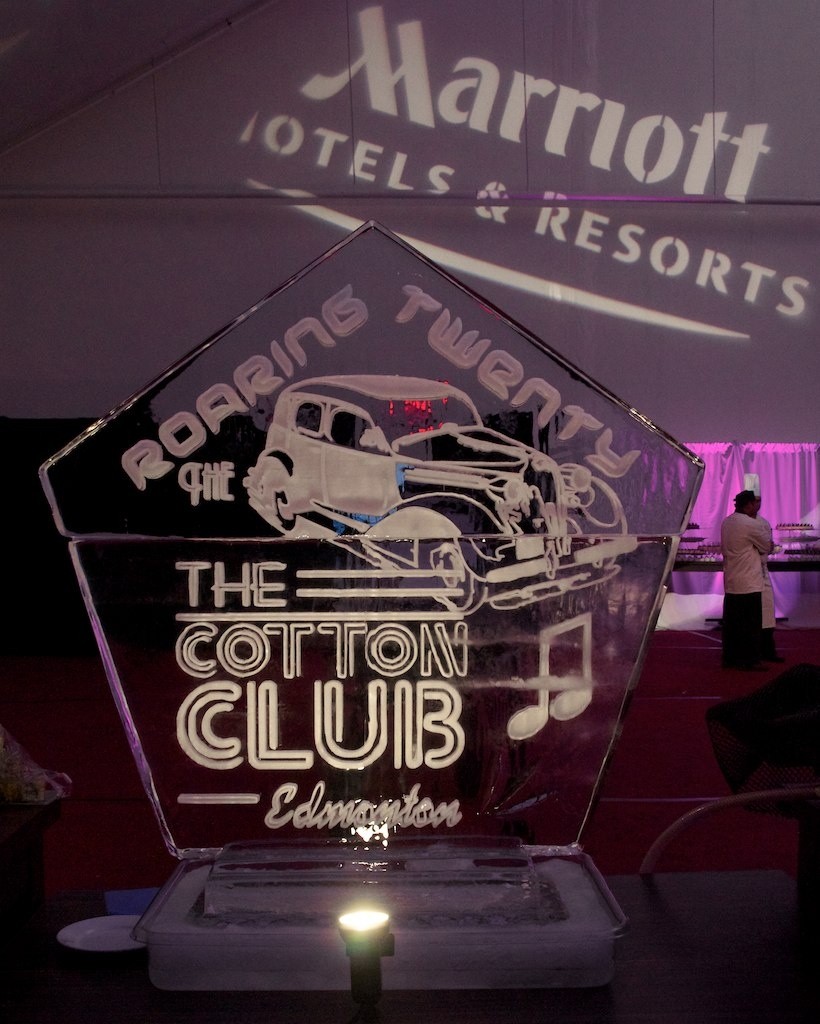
[
  {"x1": 766, "y1": 656, "x2": 785, "y2": 663},
  {"x1": 755, "y1": 665, "x2": 769, "y2": 672}
]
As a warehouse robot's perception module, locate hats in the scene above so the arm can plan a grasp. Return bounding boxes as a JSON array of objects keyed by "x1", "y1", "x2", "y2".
[
  {"x1": 744, "y1": 474, "x2": 761, "y2": 497},
  {"x1": 736, "y1": 490, "x2": 755, "y2": 506}
]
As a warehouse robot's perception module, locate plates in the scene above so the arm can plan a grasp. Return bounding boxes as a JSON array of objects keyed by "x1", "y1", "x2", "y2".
[{"x1": 57, "y1": 915, "x2": 148, "y2": 952}]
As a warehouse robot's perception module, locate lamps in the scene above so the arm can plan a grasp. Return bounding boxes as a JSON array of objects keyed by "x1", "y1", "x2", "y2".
[{"x1": 337, "y1": 910, "x2": 396, "y2": 1024}]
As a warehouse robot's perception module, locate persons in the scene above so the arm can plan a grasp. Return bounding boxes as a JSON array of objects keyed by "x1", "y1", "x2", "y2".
[{"x1": 721, "y1": 473, "x2": 787, "y2": 672}]
